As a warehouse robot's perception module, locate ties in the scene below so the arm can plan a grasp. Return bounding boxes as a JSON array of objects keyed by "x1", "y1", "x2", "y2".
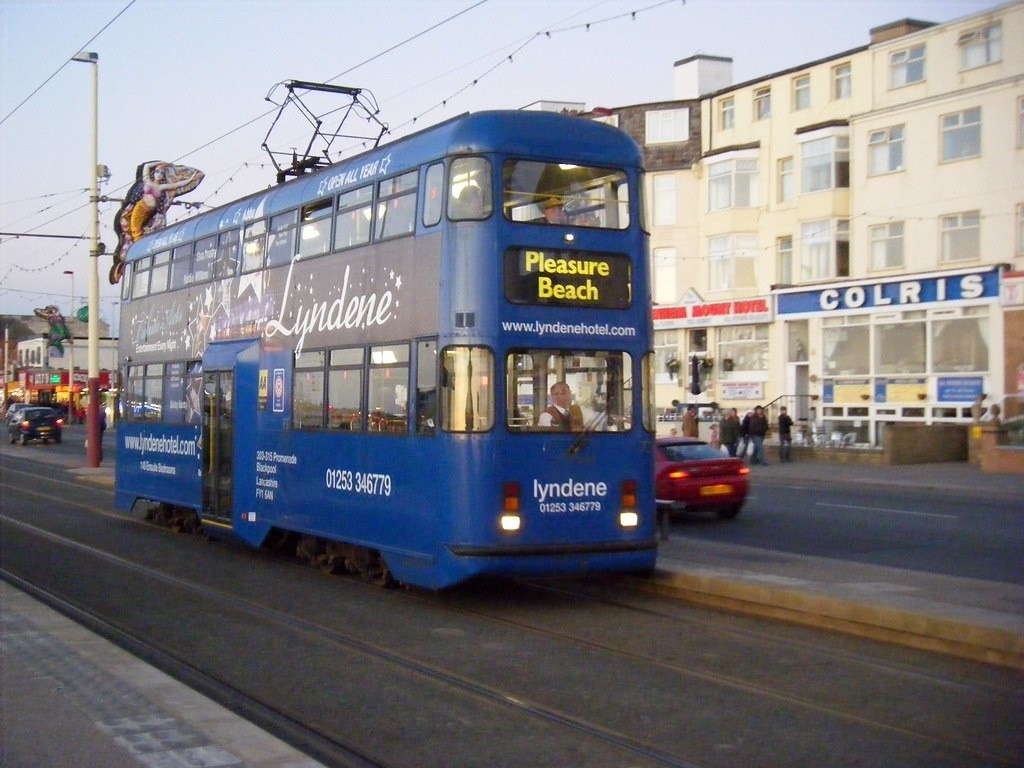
[{"x1": 564, "y1": 409, "x2": 569, "y2": 416}]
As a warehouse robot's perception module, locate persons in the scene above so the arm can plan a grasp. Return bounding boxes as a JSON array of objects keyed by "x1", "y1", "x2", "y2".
[
  {"x1": 452, "y1": 185, "x2": 487, "y2": 219},
  {"x1": 681, "y1": 402, "x2": 702, "y2": 438},
  {"x1": 532, "y1": 195, "x2": 575, "y2": 225},
  {"x1": 60, "y1": 397, "x2": 87, "y2": 425},
  {"x1": 776, "y1": 406, "x2": 795, "y2": 462},
  {"x1": 711, "y1": 405, "x2": 770, "y2": 465},
  {"x1": 535, "y1": 378, "x2": 590, "y2": 433}
]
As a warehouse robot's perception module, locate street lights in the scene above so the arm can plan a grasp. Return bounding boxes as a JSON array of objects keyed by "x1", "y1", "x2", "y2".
[{"x1": 71, "y1": 51, "x2": 102, "y2": 469}]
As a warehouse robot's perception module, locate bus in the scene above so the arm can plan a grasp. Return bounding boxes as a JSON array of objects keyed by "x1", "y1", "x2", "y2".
[{"x1": 111, "y1": 80, "x2": 663, "y2": 600}]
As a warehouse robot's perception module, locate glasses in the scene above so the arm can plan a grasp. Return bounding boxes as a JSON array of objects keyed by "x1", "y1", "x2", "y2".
[{"x1": 555, "y1": 390, "x2": 572, "y2": 394}]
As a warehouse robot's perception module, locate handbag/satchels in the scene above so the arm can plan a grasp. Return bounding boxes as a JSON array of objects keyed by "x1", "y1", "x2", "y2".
[{"x1": 100, "y1": 420, "x2": 107, "y2": 429}]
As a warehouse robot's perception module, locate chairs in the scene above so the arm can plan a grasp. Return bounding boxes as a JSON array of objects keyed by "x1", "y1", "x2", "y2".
[{"x1": 299, "y1": 411, "x2": 407, "y2": 432}]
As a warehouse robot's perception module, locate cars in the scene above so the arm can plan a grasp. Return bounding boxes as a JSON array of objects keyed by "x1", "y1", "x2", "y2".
[
  {"x1": 653, "y1": 435, "x2": 750, "y2": 521},
  {"x1": 8, "y1": 406, "x2": 64, "y2": 446},
  {"x1": 0, "y1": 400, "x2": 160, "y2": 433}
]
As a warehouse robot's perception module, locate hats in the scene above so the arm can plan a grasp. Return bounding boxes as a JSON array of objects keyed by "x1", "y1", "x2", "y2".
[{"x1": 754, "y1": 405, "x2": 764, "y2": 410}]
